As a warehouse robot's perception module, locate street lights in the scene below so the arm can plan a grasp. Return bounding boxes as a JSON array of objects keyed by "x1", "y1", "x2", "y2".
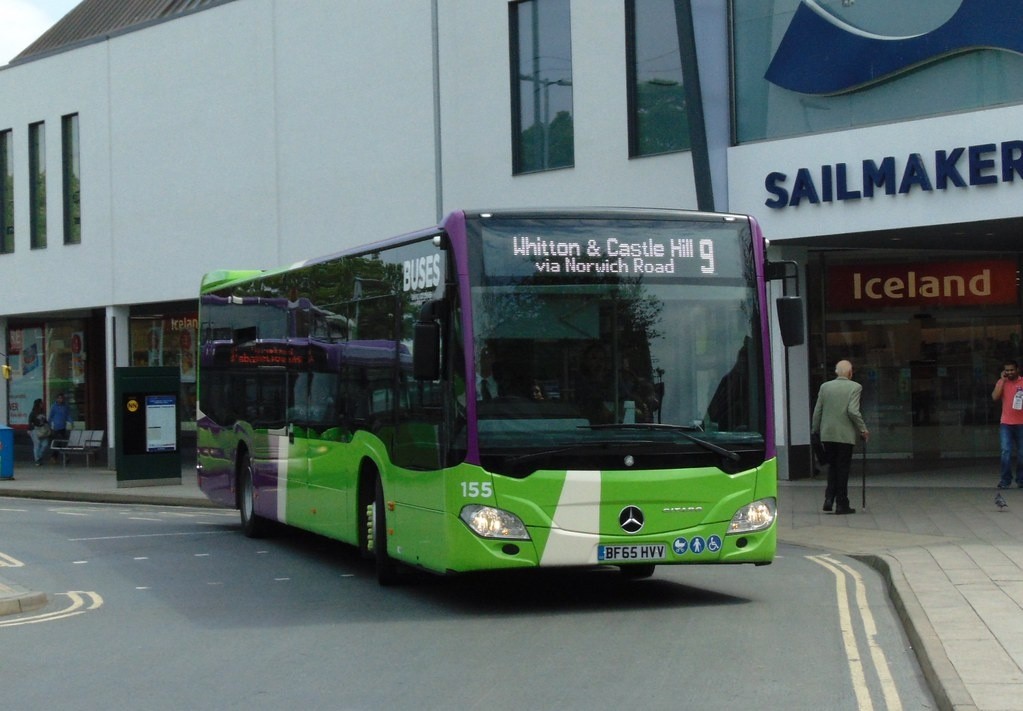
[{"x1": 520, "y1": 74, "x2": 574, "y2": 170}]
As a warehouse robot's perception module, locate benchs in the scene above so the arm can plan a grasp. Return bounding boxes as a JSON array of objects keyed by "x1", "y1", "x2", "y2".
[{"x1": 49, "y1": 429, "x2": 105, "y2": 469}]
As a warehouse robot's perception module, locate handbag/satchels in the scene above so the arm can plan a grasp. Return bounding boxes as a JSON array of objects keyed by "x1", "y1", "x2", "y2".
[
  {"x1": 811, "y1": 433, "x2": 828, "y2": 466},
  {"x1": 35, "y1": 423, "x2": 51, "y2": 438}
]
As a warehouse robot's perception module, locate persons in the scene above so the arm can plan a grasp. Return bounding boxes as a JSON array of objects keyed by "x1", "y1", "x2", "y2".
[
  {"x1": 872, "y1": 351, "x2": 901, "y2": 435},
  {"x1": 27, "y1": 399, "x2": 48, "y2": 466},
  {"x1": 811, "y1": 360, "x2": 869, "y2": 514},
  {"x1": 909, "y1": 354, "x2": 933, "y2": 426},
  {"x1": 228, "y1": 352, "x2": 285, "y2": 423},
  {"x1": 48, "y1": 393, "x2": 74, "y2": 464},
  {"x1": 575, "y1": 341, "x2": 645, "y2": 431},
  {"x1": 482, "y1": 314, "x2": 531, "y2": 397},
  {"x1": 207, "y1": 342, "x2": 252, "y2": 425},
  {"x1": 991, "y1": 360, "x2": 1023, "y2": 488},
  {"x1": 528, "y1": 379, "x2": 549, "y2": 401},
  {"x1": 294, "y1": 347, "x2": 347, "y2": 423}
]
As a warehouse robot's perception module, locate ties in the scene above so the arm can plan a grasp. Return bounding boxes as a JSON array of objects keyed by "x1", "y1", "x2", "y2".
[{"x1": 480, "y1": 379, "x2": 492, "y2": 401}]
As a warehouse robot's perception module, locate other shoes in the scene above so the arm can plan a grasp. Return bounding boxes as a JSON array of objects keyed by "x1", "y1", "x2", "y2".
[
  {"x1": 823, "y1": 501, "x2": 833, "y2": 512},
  {"x1": 1017, "y1": 480, "x2": 1023, "y2": 488},
  {"x1": 36, "y1": 460, "x2": 40, "y2": 465},
  {"x1": 835, "y1": 506, "x2": 856, "y2": 514},
  {"x1": 998, "y1": 480, "x2": 1012, "y2": 489}
]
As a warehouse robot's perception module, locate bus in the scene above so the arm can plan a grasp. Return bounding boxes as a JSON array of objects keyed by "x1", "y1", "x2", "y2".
[{"x1": 193, "y1": 203, "x2": 806, "y2": 588}]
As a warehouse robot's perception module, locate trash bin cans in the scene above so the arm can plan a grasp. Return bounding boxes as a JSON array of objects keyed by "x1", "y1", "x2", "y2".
[{"x1": 0, "y1": 424, "x2": 15, "y2": 480}]
